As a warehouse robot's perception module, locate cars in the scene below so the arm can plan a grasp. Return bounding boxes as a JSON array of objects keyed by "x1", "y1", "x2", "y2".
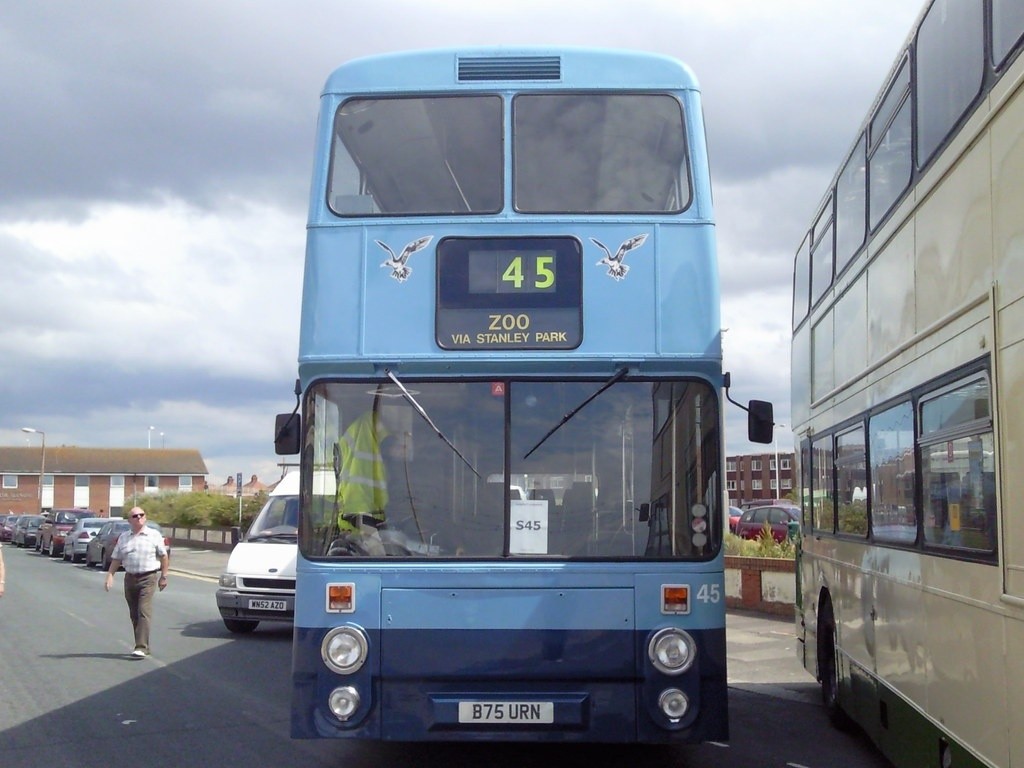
[
  {"x1": 85, "y1": 521, "x2": 170, "y2": 571},
  {"x1": 61, "y1": 517, "x2": 121, "y2": 564},
  {"x1": 0, "y1": 516, "x2": 19, "y2": 542},
  {"x1": 729, "y1": 505, "x2": 750, "y2": 533},
  {"x1": 11, "y1": 515, "x2": 47, "y2": 548}
]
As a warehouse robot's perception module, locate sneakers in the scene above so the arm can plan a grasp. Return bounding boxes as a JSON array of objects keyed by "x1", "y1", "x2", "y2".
[{"x1": 131, "y1": 646, "x2": 150, "y2": 657}]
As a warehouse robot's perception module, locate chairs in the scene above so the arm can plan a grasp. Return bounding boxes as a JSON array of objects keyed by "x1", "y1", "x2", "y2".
[{"x1": 485, "y1": 476, "x2": 600, "y2": 520}]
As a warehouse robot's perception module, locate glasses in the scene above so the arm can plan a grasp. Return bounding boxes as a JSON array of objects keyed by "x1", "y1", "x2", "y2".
[{"x1": 127, "y1": 513, "x2": 144, "y2": 519}]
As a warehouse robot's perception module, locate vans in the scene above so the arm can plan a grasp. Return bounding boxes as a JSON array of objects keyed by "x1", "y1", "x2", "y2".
[
  {"x1": 737, "y1": 498, "x2": 792, "y2": 510},
  {"x1": 216, "y1": 469, "x2": 341, "y2": 636}
]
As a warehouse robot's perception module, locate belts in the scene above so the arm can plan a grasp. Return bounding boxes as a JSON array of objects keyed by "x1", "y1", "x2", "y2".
[{"x1": 125, "y1": 568, "x2": 161, "y2": 577}]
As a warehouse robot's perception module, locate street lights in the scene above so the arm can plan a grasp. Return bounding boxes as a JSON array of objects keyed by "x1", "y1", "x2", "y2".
[
  {"x1": 160, "y1": 431, "x2": 166, "y2": 450},
  {"x1": 20, "y1": 427, "x2": 46, "y2": 516},
  {"x1": 147, "y1": 426, "x2": 155, "y2": 449}
]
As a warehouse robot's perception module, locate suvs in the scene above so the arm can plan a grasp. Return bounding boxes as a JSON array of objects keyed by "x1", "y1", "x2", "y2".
[
  {"x1": 736, "y1": 504, "x2": 801, "y2": 543},
  {"x1": 34, "y1": 509, "x2": 98, "y2": 557}
]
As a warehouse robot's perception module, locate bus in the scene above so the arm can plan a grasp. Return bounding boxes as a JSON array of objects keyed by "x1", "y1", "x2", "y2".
[
  {"x1": 269, "y1": 41, "x2": 775, "y2": 763},
  {"x1": 785, "y1": 1, "x2": 1024, "y2": 768}
]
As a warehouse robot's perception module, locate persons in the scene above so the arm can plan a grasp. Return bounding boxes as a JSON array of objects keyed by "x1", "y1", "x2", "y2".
[
  {"x1": 106, "y1": 507, "x2": 169, "y2": 658},
  {"x1": 1, "y1": 541, "x2": 6, "y2": 600}
]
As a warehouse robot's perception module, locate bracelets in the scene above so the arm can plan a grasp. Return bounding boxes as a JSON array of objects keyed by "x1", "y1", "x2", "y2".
[
  {"x1": 1, "y1": 580, "x2": 5, "y2": 584},
  {"x1": 161, "y1": 576, "x2": 168, "y2": 580}
]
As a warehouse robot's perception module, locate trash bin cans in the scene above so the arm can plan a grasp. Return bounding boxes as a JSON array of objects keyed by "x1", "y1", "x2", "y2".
[{"x1": 787, "y1": 521, "x2": 800, "y2": 540}]
